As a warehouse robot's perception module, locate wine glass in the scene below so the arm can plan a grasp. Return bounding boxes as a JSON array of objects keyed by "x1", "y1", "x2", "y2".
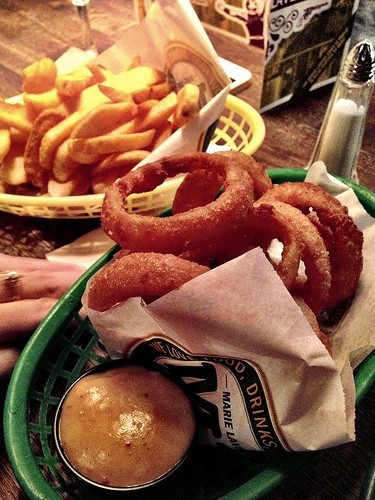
[{"x1": 47, "y1": 0, "x2": 116, "y2": 62}]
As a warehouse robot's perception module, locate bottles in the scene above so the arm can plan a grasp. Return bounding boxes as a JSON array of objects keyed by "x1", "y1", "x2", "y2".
[{"x1": 303, "y1": 39, "x2": 375, "y2": 187}]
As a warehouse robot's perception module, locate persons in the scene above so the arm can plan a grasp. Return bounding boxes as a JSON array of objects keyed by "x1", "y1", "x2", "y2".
[{"x1": 0, "y1": 252, "x2": 87, "y2": 375}]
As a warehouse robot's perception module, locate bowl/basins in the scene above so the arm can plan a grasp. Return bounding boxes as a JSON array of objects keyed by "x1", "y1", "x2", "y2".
[{"x1": 53, "y1": 356, "x2": 200, "y2": 492}]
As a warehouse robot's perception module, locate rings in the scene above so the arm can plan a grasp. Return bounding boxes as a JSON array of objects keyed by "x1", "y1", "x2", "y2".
[{"x1": 2, "y1": 272, "x2": 21, "y2": 302}]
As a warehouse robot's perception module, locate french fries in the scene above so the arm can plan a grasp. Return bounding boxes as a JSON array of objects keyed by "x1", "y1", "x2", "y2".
[{"x1": 0, "y1": 58, "x2": 201, "y2": 196}]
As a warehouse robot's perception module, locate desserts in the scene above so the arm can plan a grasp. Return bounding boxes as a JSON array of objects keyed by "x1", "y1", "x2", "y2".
[{"x1": 58, "y1": 365, "x2": 194, "y2": 488}]
